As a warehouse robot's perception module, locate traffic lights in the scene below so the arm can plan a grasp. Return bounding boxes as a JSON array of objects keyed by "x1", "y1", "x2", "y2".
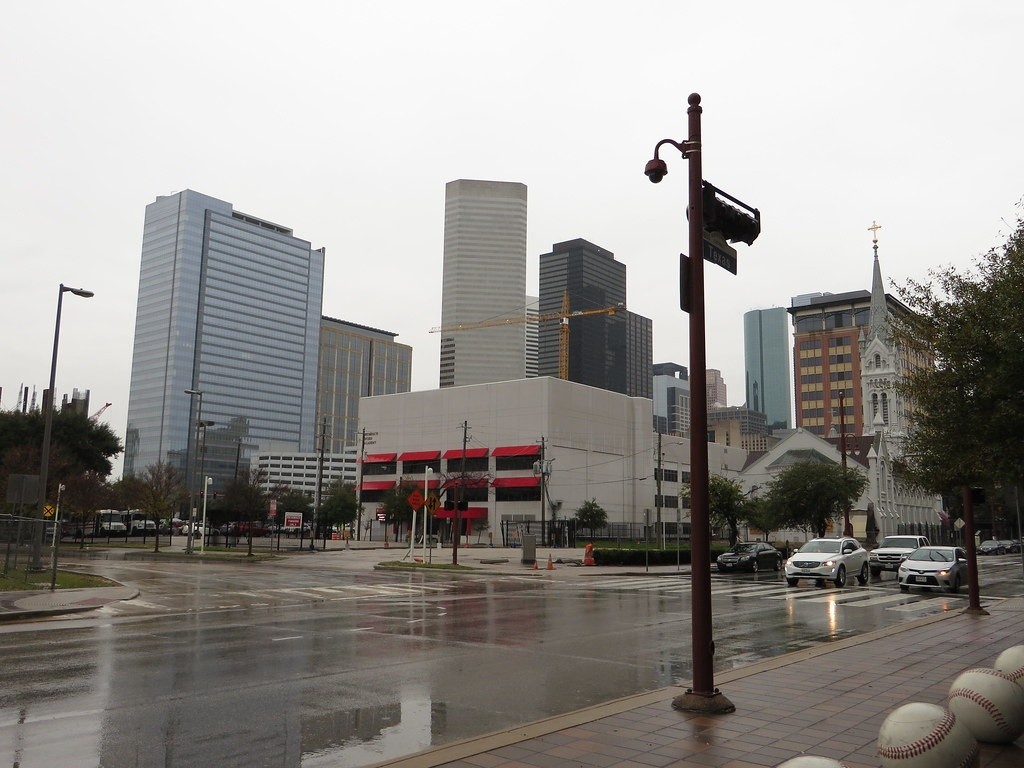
[
  {"x1": 200, "y1": 491, "x2": 204, "y2": 498},
  {"x1": 214, "y1": 491, "x2": 217, "y2": 499}
]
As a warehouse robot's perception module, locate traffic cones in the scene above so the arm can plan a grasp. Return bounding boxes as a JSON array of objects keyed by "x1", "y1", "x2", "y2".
[
  {"x1": 545, "y1": 553, "x2": 557, "y2": 570},
  {"x1": 533, "y1": 560, "x2": 539, "y2": 570}
]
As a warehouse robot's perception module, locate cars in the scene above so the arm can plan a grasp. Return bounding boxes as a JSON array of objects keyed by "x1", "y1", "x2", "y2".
[
  {"x1": 1012, "y1": 540, "x2": 1022, "y2": 553},
  {"x1": 978, "y1": 540, "x2": 1007, "y2": 555},
  {"x1": 999, "y1": 541, "x2": 1017, "y2": 554},
  {"x1": 333, "y1": 523, "x2": 355, "y2": 534},
  {"x1": 717, "y1": 542, "x2": 783, "y2": 573},
  {"x1": 267, "y1": 521, "x2": 331, "y2": 534},
  {"x1": 897, "y1": 545, "x2": 969, "y2": 593}
]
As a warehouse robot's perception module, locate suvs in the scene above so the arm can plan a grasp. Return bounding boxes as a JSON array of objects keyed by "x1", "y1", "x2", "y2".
[
  {"x1": 159, "y1": 518, "x2": 183, "y2": 535},
  {"x1": 784, "y1": 536, "x2": 870, "y2": 587},
  {"x1": 870, "y1": 535, "x2": 931, "y2": 576},
  {"x1": 183, "y1": 523, "x2": 220, "y2": 538},
  {"x1": 234, "y1": 522, "x2": 273, "y2": 538},
  {"x1": 220, "y1": 522, "x2": 237, "y2": 535}
]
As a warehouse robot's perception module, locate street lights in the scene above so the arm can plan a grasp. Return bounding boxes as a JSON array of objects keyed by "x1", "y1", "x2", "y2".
[
  {"x1": 837, "y1": 389, "x2": 850, "y2": 535},
  {"x1": 21, "y1": 284, "x2": 95, "y2": 574},
  {"x1": 644, "y1": 92, "x2": 762, "y2": 717},
  {"x1": 184, "y1": 389, "x2": 205, "y2": 553}
]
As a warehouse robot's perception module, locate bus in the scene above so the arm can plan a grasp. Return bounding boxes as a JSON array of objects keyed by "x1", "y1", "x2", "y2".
[{"x1": 46, "y1": 508, "x2": 157, "y2": 538}]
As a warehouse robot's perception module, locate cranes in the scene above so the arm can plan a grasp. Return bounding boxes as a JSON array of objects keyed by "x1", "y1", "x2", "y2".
[{"x1": 427, "y1": 288, "x2": 627, "y2": 380}]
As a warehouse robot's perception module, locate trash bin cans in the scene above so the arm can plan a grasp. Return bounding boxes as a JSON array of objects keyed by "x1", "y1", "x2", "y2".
[{"x1": 521, "y1": 535, "x2": 536, "y2": 564}]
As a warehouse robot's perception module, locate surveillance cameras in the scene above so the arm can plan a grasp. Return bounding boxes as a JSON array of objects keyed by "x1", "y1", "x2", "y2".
[{"x1": 645, "y1": 160, "x2": 668, "y2": 183}]
